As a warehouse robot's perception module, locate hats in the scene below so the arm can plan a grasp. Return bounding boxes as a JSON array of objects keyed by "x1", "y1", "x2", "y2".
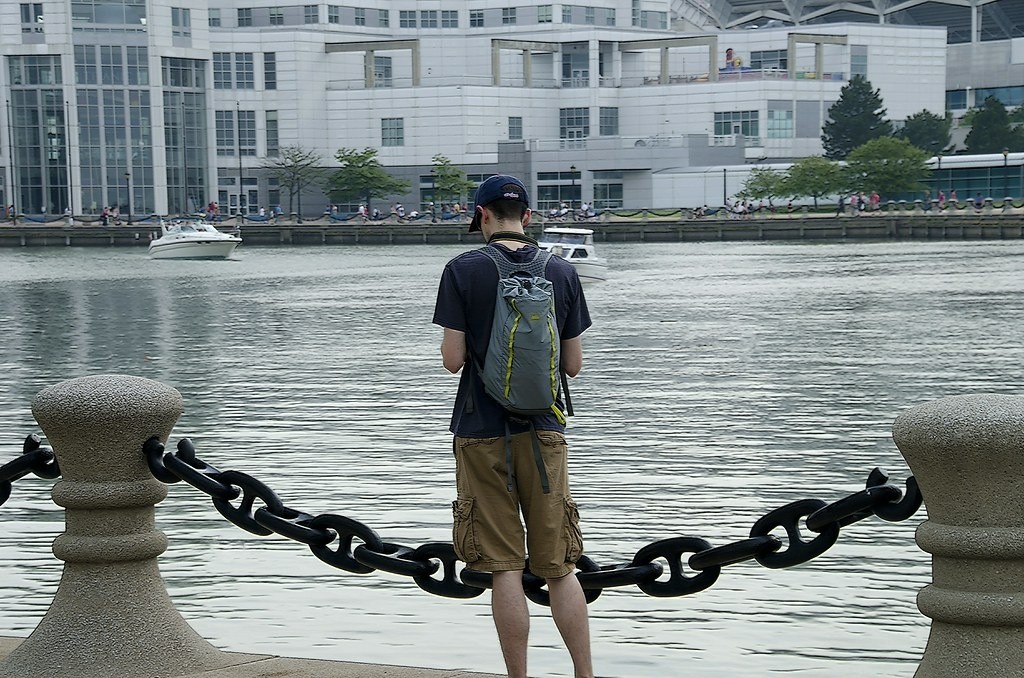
[{"x1": 467, "y1": 173, "x2": 530, "y2": 233}]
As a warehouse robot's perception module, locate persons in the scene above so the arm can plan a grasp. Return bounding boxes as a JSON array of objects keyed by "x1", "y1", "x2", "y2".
[
  {"x1": 204, "y1": 201, "x2": 219, "y2": 221},
  {"x1": 9, "y1": 205, "x2": 14, "y2": 217},
  {"x1": 432, "y1": 175, "x2": 592, "y2": 678},
  {"x1": 922, "y1": 189, "x2": 934, "y2": 214},
  {"x1": 428, "y1": 201, "x2": 468, "y2": 223},
  {"x1": 849, "y1": 190, "x2": 880, "y2": 216},
  {"x1": 786, "y1": 200, "x2": 793, "y2": 219},
  {"x1": 937, "y1": 191, "x2": 947, "y2": 213},
  {"x1": 950, "y1": 189, "x2": 957, "y2": 199},
  {"x1": 581, "y1": 202, "x2": 589, "y2": 212},
  {"x1": 259, "y1": 205, "x2": 282, "y2": 217},
  {"x1": 588, "y1": 202, "x2": 593, "y2": 211},
  {"x1": 551, "y1": 200, "x2": 568, "y2": 222},
  {"x1": 326, "y1": 205, "x2": 338, "y2": 214},
  {"x1": 974, "y1": 192, "x2": 983, "y2": 205},
  {"x1": 359, "y1": 204, "x2": 379, "y2": 219},
  {"x1": 836, "y1": 195, "x2": 846, "y2": 217},
  {"x1": 102, "y1": 207, "x2": 121, "y2": 226},
  {"x1": 390, "y1": 201, "x2": 418, "y2": 221},
  {"x1": 689, "y1": 196, "x2": 777, "y2": 221}
]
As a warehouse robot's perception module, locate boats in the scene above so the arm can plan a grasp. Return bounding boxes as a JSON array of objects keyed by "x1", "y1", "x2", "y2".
[
  {"x1": 145, "y1": 222, "x2": 243, "y2": 260},
  {"x1": 534, "y1": 227, "x2": 611, "y2": 284}
]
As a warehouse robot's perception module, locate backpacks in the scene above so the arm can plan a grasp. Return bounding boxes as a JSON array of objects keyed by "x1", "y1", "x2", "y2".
[{"x1": 464, "y1": 244, "x2": 575, "y2": 497}]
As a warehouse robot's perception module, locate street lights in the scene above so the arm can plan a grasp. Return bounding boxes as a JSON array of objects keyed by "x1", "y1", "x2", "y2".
[
  {"x1": 430, "y1": 168, "x2": 437, "y2": 223},
  {"x1": 1002, "y1": 146, "x2": 1010, "y2": 199},
  {"x1": 570, "y1": 164, "x2": 577, "y2": 221},
  {"x1": 937, "y1": 153, "x2": 943, "y2": 191},
  {"x1": 124, "y1": 172, "x2": 134, "y2": 226}
]
